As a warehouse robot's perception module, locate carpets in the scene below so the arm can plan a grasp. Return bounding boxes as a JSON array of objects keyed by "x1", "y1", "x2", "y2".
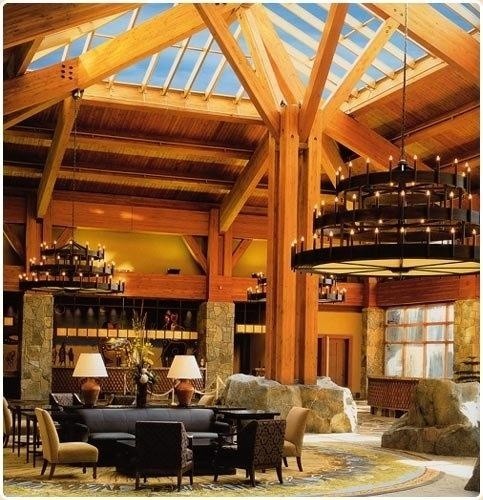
[{"x1": 3, "y1": 438, "x2": 447, "y2": 497}]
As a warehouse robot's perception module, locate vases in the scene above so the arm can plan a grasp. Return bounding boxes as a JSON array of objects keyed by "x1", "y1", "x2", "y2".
[{"x1": 135, "y1": 383, "x2": 147, "y2": 407}]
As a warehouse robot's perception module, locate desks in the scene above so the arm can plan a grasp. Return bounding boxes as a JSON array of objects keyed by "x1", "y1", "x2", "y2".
[
  {"x1": 116, "y1": 441, "x2": 237, "y2": 477},
  {"x1": 219, "y1": 410, "x2": 281, "y2": 442}
]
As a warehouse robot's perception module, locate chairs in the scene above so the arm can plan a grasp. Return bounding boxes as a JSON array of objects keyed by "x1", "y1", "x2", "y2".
[
  {"x1": 213, "y1": 419, "x2": 287, "y2": 487},
  {"x1": 35, "y1": 407, "x2": 99, "y2": 479},
  {"x1": 133, "y1": 420, "x2": 193, "y2": 492},
  {"x1": 48, "y1": 392, "x2": 84, "y2": 423},
  {"x1": 262, "y1": 406, "x2": 308, "y2": 472}
]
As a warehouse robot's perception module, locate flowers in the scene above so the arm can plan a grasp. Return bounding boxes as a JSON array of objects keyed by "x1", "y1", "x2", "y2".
[{"x1": 105, "y1": 305, "x2": 161, "y2": 396}]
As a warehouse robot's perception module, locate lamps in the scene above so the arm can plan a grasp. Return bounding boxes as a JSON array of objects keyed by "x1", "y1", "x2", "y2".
[
  {"x1": 165, "y1": 354, "x2": 202, "y2": 407},
  {"x1": 70, "y1": 353, "x2": 108, "y2": 406},
  {"x1": 243, "y1": 270, "x2": 347, "y2": 306},
  {"x1": 287, "y1": 3, "x2": 479, "y2": 285},
  {"x1": 20, "y1": 86, "x2": 126, "y2": 292}
]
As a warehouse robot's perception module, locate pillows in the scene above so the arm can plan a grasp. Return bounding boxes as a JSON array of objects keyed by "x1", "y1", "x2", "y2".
[{"x1": 197, "y1": 395, "x2": 215, "y2": 406}]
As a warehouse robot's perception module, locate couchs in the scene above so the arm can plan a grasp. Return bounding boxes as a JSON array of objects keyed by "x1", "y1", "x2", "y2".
[{"x1": 59, "y1": 409, "x2": 231, "y2": 468}]
